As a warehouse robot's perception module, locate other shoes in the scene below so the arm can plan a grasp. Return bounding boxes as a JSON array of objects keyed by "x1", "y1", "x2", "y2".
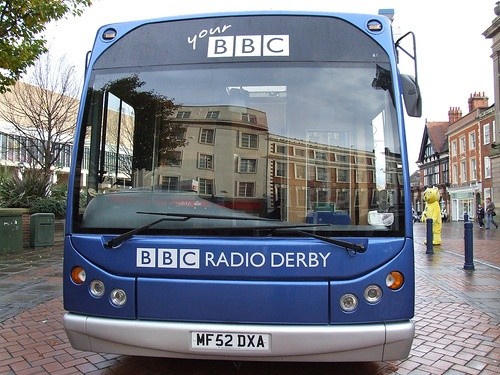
[
  {"x1": 483, "y1": 224, "x2": 486, "y2": 228},
  {"x1": 480, "y1": 227, "x2": 482, "y2": 229},
  {"x1": 496, "y1": 224, "x2": 498, "y2": 229},
  {"x1": 485, "y1": 227, "x2": 490, "y2": 229}
]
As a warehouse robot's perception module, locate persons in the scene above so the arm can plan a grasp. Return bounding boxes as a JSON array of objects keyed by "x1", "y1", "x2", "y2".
[
  {"x1": 476, "y1": 204, "x2": 485, "y2": 228},
  {"x1": 485, "y1": 198, "x2": 498, "y2": 229}
]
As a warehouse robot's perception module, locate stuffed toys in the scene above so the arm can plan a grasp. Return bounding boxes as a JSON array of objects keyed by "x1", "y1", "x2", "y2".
[{"x1": 420, "y1": 187, "x2": 442, "y2": 246}]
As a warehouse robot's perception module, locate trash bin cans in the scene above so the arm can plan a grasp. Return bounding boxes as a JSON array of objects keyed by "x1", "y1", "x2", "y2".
[
  {"x1": 29, "y1": 212, "x2": 55, "y2": 248},
  {"x1": 0, "y1": 215, "x2": 24, "y2": 254}
]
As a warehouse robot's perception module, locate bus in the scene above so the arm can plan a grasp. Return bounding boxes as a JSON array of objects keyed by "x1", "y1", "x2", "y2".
[{"x1": 60, "y1": 8, "x2": 424, "y2": 364}]
